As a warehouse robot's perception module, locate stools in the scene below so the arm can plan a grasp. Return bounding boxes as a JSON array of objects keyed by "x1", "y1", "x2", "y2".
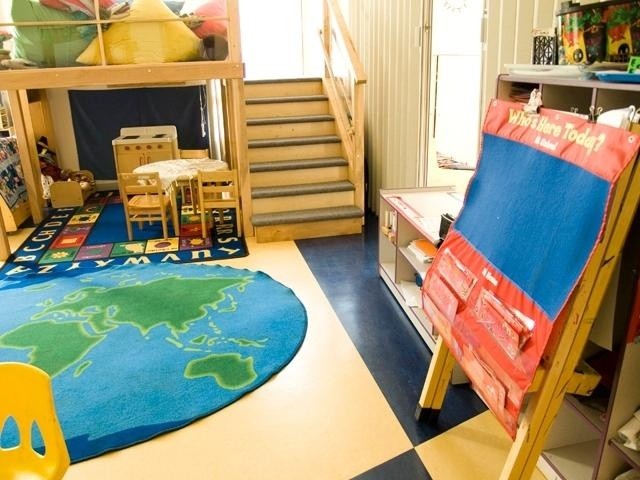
[{"x1": 47, "y1": 170, "x2": 95, "y2": 210}]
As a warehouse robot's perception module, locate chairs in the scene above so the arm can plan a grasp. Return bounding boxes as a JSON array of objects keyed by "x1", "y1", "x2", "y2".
[{"x1": 117, "y1": 149, "x2": 242, "y2": 238}]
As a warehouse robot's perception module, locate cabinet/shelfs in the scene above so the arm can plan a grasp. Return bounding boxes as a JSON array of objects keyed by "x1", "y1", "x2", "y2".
[{"x1": 113, "y1": 139, "x2": 179, "y2": 198}]
